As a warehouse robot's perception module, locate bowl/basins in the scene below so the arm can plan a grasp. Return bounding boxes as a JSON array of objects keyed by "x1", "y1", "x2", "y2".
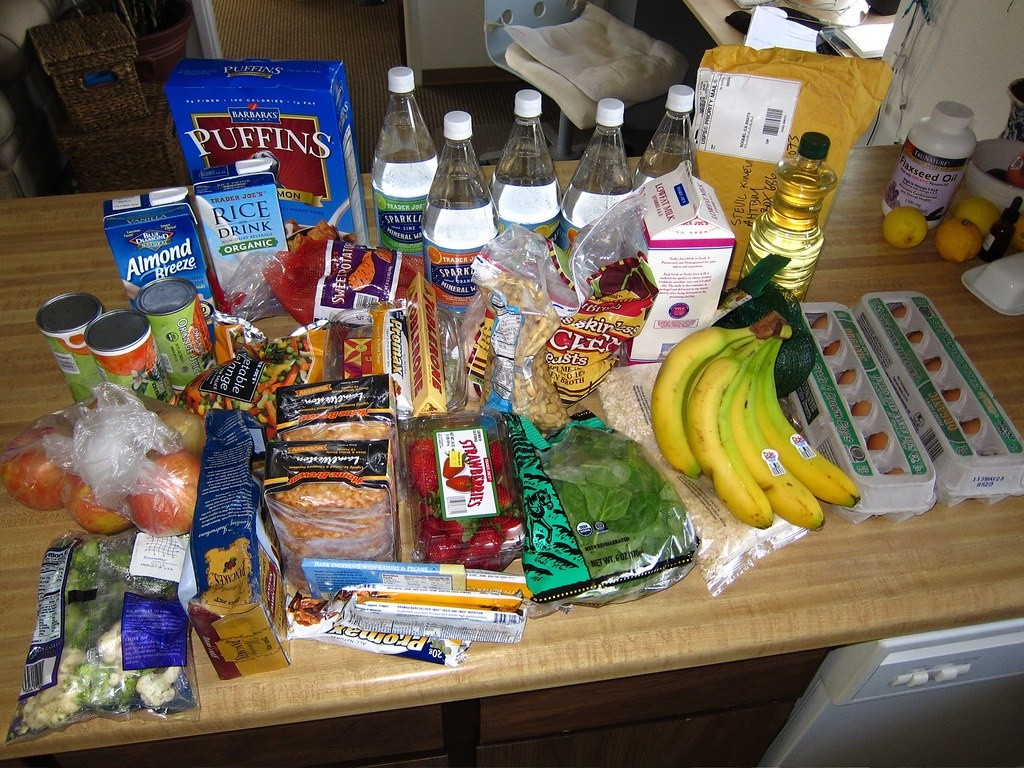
[
  {"x1": 399, "y1": 410, "x2": 528, "y2": 571},
  {"x1": 323, "y1": 305, "x2": 470, "y2": 410},
  {"x1": 967, "y1": 140, "x2": 1024, "y2": 213}
]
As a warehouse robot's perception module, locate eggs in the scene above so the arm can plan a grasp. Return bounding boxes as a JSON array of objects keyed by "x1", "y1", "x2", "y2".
[{"x1": 812, "y1": 305, "x2": 981, "y2": 475}]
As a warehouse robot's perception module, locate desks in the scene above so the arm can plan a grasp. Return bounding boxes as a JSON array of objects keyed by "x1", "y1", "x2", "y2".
[
  {"x1": 0, "y1": 144, "x2": 1024, "y2": 768},
  {"x1": 683, "y1": 0, "x2": 900, "y2": 61}
]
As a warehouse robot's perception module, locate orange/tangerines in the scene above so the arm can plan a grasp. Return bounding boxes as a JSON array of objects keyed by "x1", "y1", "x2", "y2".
[{"x1": 883, "y1": 197, "x2": 1024, "y2": 263}]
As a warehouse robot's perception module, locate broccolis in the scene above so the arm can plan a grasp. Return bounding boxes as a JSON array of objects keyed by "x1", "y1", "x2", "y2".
[{"x1": 15, "y1": 538, "x2": 181, "y2": 731}]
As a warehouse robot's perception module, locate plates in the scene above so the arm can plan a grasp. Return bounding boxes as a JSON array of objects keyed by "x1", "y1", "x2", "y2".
[{"x1": 961, "y1": 253, "x2": 1024, "y2": 316}]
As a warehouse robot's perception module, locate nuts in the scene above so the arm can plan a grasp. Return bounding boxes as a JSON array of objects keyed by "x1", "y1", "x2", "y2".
[{"x1": 460, "y1": 272, "x2": 570, "y2": 432}]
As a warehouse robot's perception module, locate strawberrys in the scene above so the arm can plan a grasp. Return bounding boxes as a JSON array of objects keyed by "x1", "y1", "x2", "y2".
[{"x1": 407, "y1": 429, "x2": 525, "y2": 572}]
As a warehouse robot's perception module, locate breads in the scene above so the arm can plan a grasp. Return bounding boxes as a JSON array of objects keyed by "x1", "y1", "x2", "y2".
[{"x1": 271, "y1": 420, "x2": 396, "y2": 593}]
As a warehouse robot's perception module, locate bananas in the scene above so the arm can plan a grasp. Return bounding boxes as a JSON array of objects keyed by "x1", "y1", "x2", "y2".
[{"x1": 650, "y1": 308, "x2": 860, "y2": 532}]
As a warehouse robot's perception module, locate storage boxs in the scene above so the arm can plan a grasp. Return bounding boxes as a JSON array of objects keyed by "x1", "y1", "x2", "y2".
[
  {"x1": 54, "y1": 83, "x2": 189, "y2": 193},
  {"x1": 32, "y1": 14, "x2": 152, "y2": 133},
  {"x1": 165, "y1": 60, "x2": 369, "y2": 246}
]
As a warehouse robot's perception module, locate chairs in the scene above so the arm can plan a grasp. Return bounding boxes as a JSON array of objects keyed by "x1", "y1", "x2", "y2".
[{"x1": 479, "y1": 0, "x2": 696, "y2": 165}]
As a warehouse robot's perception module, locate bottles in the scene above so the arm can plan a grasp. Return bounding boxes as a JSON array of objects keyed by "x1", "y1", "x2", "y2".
[
  {"x1": 632, "y1": 85, "x2": 700, "y2": 186},
  {"x1": 977, "y1": 197, "x2": 1021, "y2": 259},
  {"x1": 557, "y1": 97, "x2": 634, "y2": 256},
  {"x1": 371, "y1": 66, "x2": 439, "y2": 258},
  {"x1": 738, "y1": 132, "x2": 837, "y2": 299},
  {"x1": 420, "y1": 111, "x2": 500, "y2": 318},
  {"x1": 881, "y1": 101, "x2": 976, "y2": 233},
  {"x1": 486, "y1": 90, "x2": 562, "y2": 244}
]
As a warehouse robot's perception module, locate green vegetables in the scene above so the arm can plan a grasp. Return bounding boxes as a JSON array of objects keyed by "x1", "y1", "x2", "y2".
[{"x1": 534, "y1": 427, "x2": 694, "y2": 583}]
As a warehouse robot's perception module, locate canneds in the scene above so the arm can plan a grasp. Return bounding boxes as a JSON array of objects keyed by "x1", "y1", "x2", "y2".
[{"x1": 35, "y1": 277, "x2": 215, "y2": 409}]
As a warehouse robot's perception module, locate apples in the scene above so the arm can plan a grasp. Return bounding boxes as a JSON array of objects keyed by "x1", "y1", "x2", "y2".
[{"x1": 0, "y1": 404, "x2": 205, "y2": 538}]
